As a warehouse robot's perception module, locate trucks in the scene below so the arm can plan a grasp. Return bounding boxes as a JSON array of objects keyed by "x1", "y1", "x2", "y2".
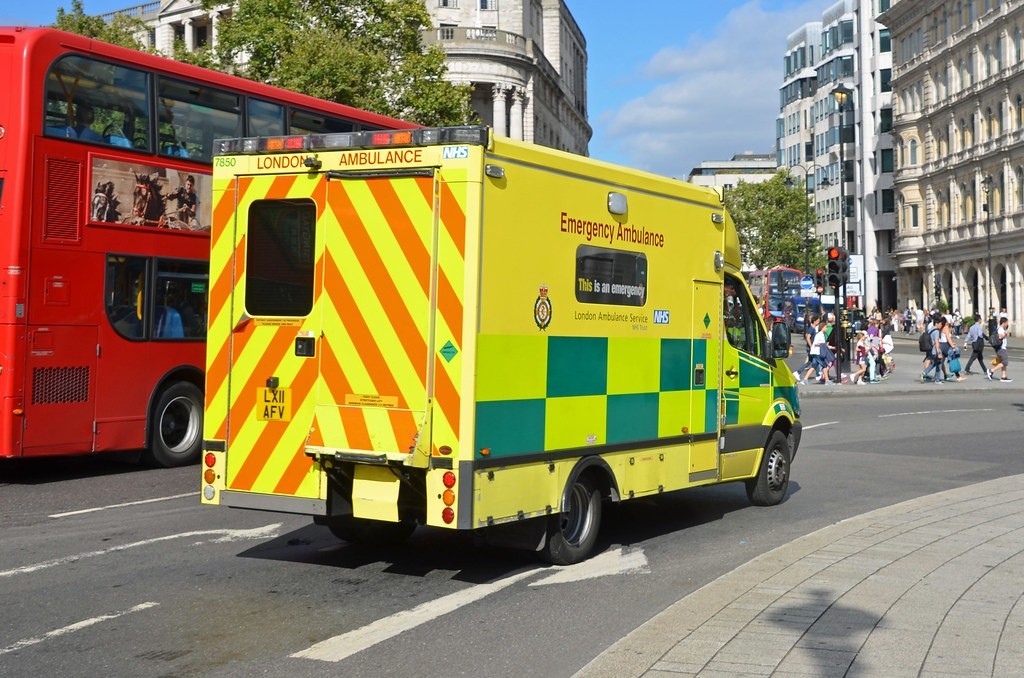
[{"x1": 795, "y1": 296, "x2": 819, "y2": 332}]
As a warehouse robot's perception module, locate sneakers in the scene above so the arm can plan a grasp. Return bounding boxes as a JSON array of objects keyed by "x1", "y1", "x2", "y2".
[
  {"x1": 987, "y1": 368, "x2": 994, "y2": 381},
  {"x1": 1000, "y1": 376, "x2": 1013, "y2": 382}
]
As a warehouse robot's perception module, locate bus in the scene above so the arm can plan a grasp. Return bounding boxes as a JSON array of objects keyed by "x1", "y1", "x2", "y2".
[
  {"x1": 0, "y1": 27, "x2": 430, "y2": 470},
  {"x1": 748, "y1": 268, "x2": 804, "y2": 333}
]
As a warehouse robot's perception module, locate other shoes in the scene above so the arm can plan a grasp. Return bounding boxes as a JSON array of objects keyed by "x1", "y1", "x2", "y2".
[
  {"x1": 964, "y1": 370, "x2": 971, "y2": 374},
  {"x1": 856, "y1": 379, "x2": 868, "y2": 385},
  {"x1": 801, "y1": 379, "x2": 808, "y2": 386},
  {"x1": 920, "y1": 372, "x2": 925, "y2": 384},
  {"x1": 862, "y1": 370, "x2": 890, "y2": 381},
  {"x1": 819, "y1": 371, "x2": 824, "y2": 374},
  {"x1": 841, "y1": 378, "x2": 849, "y2": 382},
  {"x1": 935, "y1": 380, "x2": 944, "y2": 384},
  {"x1": 924, "y1": 376, "x2": 935, "y2": 382},
  {"x1": 841, "y1": 372, "x2": 847, "y2": 378},
  {"x1": 870, "y1": 379, "x2": 880, "y2": 383},
  {"x1": 816, "y1": 374, "x2": 825, "y2": 381},
  {"x1": 943, "y1": 373, "x2": 954, "y2": 381},
  {"x1": 957, "y1": 376, "x2": 967, "y2": 381},
  {"x1": 793, "y1": 370, "x2": 800, "y2": 381},
  {"x1": 825, "y1": 380, "x2": 836, "y2": 386},
  {"x1": 850, "y1": 373, "x2": 858, "y2": 384}
]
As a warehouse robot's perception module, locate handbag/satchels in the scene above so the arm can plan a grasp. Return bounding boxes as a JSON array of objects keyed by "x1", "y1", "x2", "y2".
[
  {"x1": 949, "y1": 353, "x2": 962, "y2": 372},
  {"x1": 990, "y1": 355, "x2": 1002, "y2": 366}
]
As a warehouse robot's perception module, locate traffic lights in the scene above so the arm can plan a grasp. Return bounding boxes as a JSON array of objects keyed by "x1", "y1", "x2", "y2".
[
  {"x1": 814, "y1": 267, "x2": 824, "y2": 295},
  {"x1": 934, "y1": 284, "x2": 941, "y2": 296},
  {"x1": 828, "y1": 247, "x2": 842, "y2": 288},
  {"x1": 840, "y1": 249, "x2": 851, "y2": 284}
]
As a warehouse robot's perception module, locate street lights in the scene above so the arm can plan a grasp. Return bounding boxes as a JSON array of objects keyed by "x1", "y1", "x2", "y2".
[
  {"x1": 829, "y1": 79, "x2": 851, "y2": 310},
  {"x1": 981, "y1": 176, "x2": 994, "y2": 321},
  {"x1": 782, "y1": 163, "x2": 831, "y2": 277}
]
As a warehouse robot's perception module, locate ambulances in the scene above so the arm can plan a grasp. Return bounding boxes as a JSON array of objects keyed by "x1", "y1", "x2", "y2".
[{"x1": 201, "y1": 125, "x2": 804, "y2": 567}]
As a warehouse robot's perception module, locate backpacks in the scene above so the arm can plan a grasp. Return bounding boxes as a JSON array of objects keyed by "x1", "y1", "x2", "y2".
[
  {"x1": 918, "y1": 328, "x2": 939, "y2": 352},
  {"x1": 989, "y1": 327, "x2": 1003, "y2": 350}
]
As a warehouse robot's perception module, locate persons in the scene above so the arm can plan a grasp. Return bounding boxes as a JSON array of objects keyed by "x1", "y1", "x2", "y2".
[
  {"x1": 902, "y1": 307, "x2": 966, "y2": 384},
  {"x1": 993, "y1": 307, "x2": 1007, "y2": 328},
  {"x1": 964, "y1": 315, "x2": 989, "y2": 376},
  {"x1": 166, "y1": 176, "x2": 197, "y2": 223},
  {"x1": 849, "y1": 309, "x2": 900, "y2": 385},
  {"x1": 987, "y1": 317, "x2": 1013, "y2": 382},
  {"x1": 73, "y1": 104, "x2": 103, "y2": 141},
  {"x1": 793, "y1": 309, "x2": 850, "y2": 386},
  {"x1": 130, "y1": 279, "x2": 184, "y2": 338}
]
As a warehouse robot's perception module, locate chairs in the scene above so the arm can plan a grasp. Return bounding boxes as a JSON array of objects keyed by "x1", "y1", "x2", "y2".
[
  {"x1": 45, "y1": 120, "x2": 190, "y2": 158},
  {"x1": 128, "y1": 305, "x2": 185, "y2": 338}
]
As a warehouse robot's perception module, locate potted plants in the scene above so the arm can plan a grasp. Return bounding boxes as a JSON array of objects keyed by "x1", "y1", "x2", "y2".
[{"x1": 962, "y1": 317, "x2": 976, "y2": 332}]
